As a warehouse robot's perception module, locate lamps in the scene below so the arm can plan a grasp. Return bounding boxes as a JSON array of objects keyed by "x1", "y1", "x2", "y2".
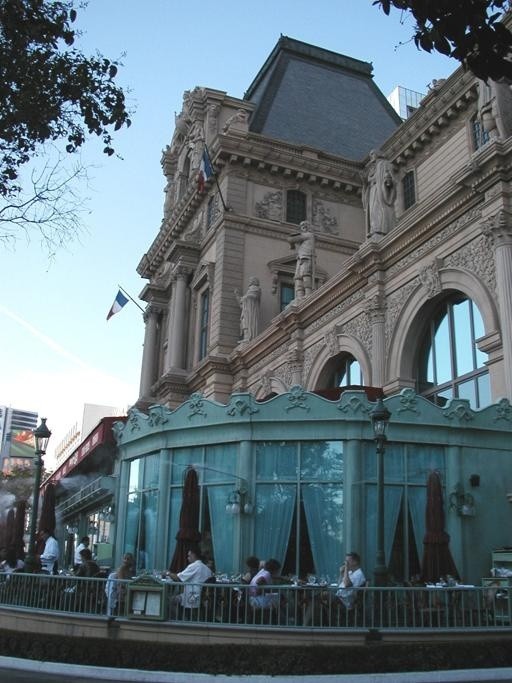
[
  {"x1": 98, "y1": 504, "x2": 115, "y2": 522},
  {"x1": 67, "y1": 521, "x2": 79, "y2": 534},
  {"x1": 447, "y1": 490, "x2": 476, "y2": 518},
  {"x1": 88, "y1": 522, "x2": 98, "y2": 535},
  {"x1": 225, "y1": 488, "x2": 255, "y2": 515}
]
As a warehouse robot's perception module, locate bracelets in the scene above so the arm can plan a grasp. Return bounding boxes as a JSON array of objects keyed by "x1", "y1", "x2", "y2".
[{"x1": 166, "y1": 570, "x2": 171, "y2": 575}]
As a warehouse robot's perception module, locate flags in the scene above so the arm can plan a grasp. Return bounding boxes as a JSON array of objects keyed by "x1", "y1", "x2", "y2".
[{"x1": 106, "y1": 288, "x2": 129, "y2": 318}]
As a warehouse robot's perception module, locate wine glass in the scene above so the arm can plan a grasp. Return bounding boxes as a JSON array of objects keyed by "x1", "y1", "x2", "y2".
[
  {"x1": 210, "y1": 568, "x2": 243, "y2": 582},
  {"x1": 139, "y1": 567, "x2": 169, "y2": 580},
  {"x1": 287, "y1": 571, "x2": 331, "y2": 587}
]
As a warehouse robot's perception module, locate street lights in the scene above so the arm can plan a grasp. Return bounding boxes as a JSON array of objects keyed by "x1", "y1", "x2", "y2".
[
  {"x1": 21, "y1": 416, "x2": 53, "y2": 595},
  {"x1": 370, "y1": 396, "x2": 391, "y2": 621}
]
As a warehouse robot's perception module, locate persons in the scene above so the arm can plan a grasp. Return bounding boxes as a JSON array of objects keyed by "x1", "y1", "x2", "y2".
[
  {"x1": 162, "y1": 548, "x2": 296, "y2": 625},
  {"x1": 0, "y1": 545, "x2": 25, "y2": 585},
  {"x1": 284, "y1": 219, "x2": 317, "y2": 298},
  {"x1": 356, "y1": 146, "x2": 399, "y2": 238},
  {"x1": 300, "y1": 550, "x2": 365, "y2": 626},
  {"x1": 116, "y1": 551, "x2": 134, "y2": 600},
  {"x1": 73, "y1": 535, "x2": 90, "y2": 565},
  {"x1": 36, "y1": 527, "x2": 60, "y2": 572},
  {"x1": 231, "y1": 273, "x2": 261, "y2": 342},
  {"x1": 70, "y1": 548, "x2": 100, "y2": 589}
]
